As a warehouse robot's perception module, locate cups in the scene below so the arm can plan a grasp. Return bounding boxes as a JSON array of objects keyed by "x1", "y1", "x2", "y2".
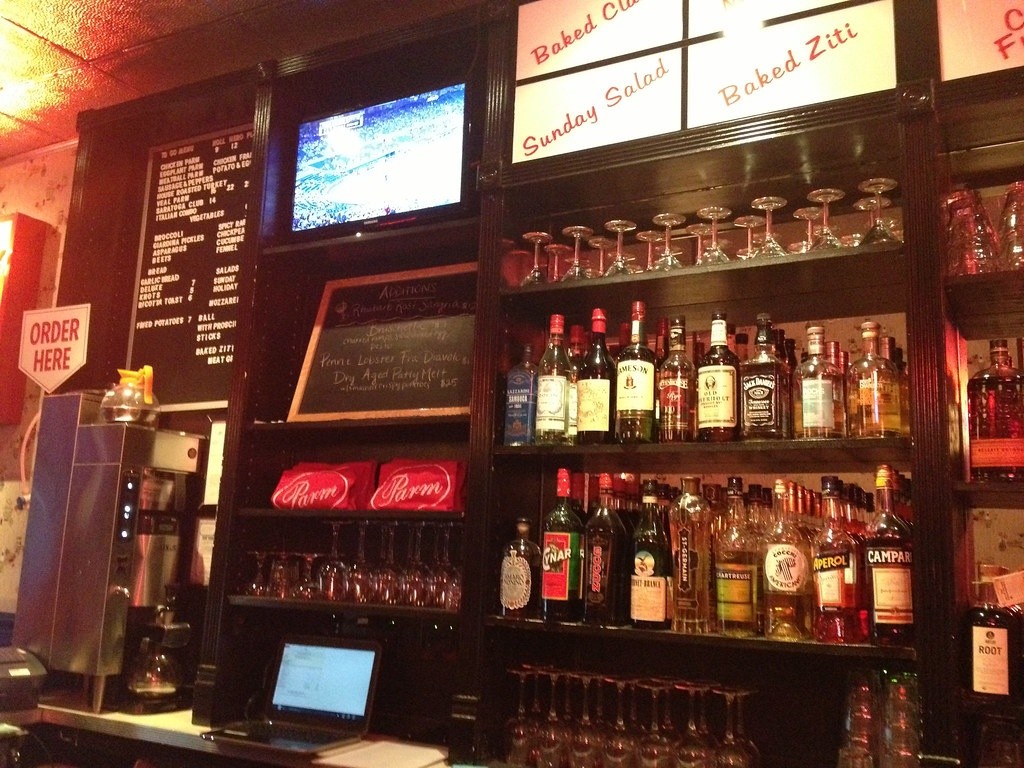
[{"x1": 946, "y1": 181, "x2": 1024, "y2": 271}]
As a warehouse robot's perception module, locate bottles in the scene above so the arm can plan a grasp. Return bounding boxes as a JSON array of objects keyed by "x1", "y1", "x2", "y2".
[
  {"x1": 964, "y1": 565, "x2": 1023, "y2": 705},
  {"x1": 500, "y1": 517, "x2": 543, "y2": 617},
  {"x1": 698, "y1": 307, "x2": 797, "y2": 440},
  {"x1": 447, "y1": 695, "x2": 481, "y2": 764},
  {"x1": 534, "y1": 314, "x2": 593, "y2": 447},
  {"x1": 713, "y1": 476, "x2": 873, "y2": 645},
  {"x1": 191, "y1": 664, "x2": 217, "y2": 726},
  {"x1": 540, "y1": 469, "x2": 727, "y2": 635},
  {"x1": 660, "y1": 315, "x2": 705, "y2": 443},
  {"x1": 966, "y1": 339, "x2": 1024, "y2": 480},
  {"x1": 863, "y1": 465, "x2": 912, "y2": 648},
  {"x1": 504, "y1": 343, "x2": 538, "y2": 446},
  {"x1": 578, "y1": 302, "x2": 668, "y2": 444},
  {"x1": 790, "y1": 322, "x2": 910, "y2": 439}
]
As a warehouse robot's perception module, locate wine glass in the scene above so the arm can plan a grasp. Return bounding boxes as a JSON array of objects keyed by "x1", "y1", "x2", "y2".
[
  {"x1": 788, "y1": 188, "x2": 845, "y2": 253},
  {"x1": 241, "y1": 552, "x2": 326, "y2": 599},
  {"x1": 313, "y1": 520, "x2": 464, "y2": 609},
  {"x1": 505, "y1": 665, "x2": 756, "y2": 768},
  {"x1": 841, "y1": 178, "x2": 902, "y2": 246},
  {"x1": 518, "y1": 185, "x2": 788, "y2": 287}
]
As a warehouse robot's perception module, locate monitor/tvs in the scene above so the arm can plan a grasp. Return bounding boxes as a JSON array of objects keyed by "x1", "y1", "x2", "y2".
[{"x1": 285, "y1": 71, "x2": 485, "y2": 245}]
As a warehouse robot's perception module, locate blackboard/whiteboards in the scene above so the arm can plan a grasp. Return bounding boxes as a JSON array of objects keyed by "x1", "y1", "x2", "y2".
[
  {"x1": 285, "y1": 261, "x2": 480, "y2": 422},
  {"x1": 126, "y1": 120, "x2": 252, "y2": 412}
]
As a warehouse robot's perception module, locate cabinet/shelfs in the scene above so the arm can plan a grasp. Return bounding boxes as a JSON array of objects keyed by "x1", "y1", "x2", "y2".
[{"x1": 54, "y1": 0, "x2": 1024, "y2": 767}]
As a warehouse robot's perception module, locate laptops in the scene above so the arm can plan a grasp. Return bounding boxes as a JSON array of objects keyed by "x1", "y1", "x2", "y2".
[{"x1": 201, "y1": 634, "x2": 381, "y2": 756}]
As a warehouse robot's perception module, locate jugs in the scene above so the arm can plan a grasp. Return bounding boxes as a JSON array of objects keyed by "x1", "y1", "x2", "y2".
[{"x1": 100, "y1": 365, "x2": 160, "y2": 424}]
